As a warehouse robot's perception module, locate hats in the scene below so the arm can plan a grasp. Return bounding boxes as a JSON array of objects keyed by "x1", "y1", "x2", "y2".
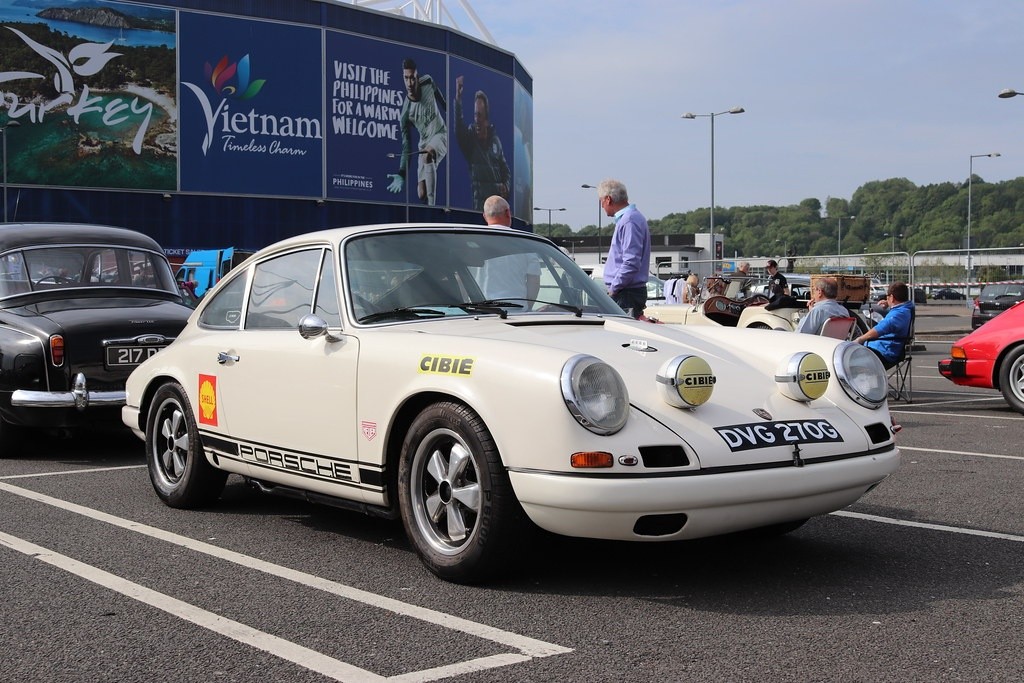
[{"x1": 763, "y1": 260, "x2": 777, "y2": 269}]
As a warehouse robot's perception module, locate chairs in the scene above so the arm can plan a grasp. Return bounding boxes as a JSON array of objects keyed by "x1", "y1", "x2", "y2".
[
  {"x1": 815, "y1": 316, "x2": 857, "y2": 342},
  {"x1": 866, "y1": 305, "x2": 916, "y2": 403},
  {"x1": 401, "y1": 268, "x2": 464, "y2": 306}
]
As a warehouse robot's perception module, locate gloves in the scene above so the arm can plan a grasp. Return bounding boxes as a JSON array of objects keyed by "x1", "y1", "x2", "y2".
[{"x1": 386, "y1": 174, "x2": 404, "y2": 193}]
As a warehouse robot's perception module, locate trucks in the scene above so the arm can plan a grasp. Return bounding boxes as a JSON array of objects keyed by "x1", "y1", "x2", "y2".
[{"x1": 173, "y1": 245, "x2": 264, "y2": 301}]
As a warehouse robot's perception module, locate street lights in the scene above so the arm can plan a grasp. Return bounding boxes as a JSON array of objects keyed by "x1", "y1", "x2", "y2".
[
  {"x1": 580, "y1": 183, "x2": 602, "y2": 264},
  {"x1": 883, "y1": 233, "x2": 903, "y2": 282},
  {"x1": 680, "y1": 106, "x2": 746, "y2": 275},
  {"x1": 966, "y1": 152, "x2": 1002, "y2": 309},
  {"x1": 533, "y1": 206, "x2": 567, "y2": 240},
  {"x1": 775, "y1": 239, "x2": 787, "y2": 272},
  {"x1": 821, "y1": 215, "x2": 856, "y2": 273}
]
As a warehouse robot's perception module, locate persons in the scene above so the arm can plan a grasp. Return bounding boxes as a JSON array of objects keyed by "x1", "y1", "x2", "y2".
[
  {"x1": 794, "y1": 278, "x2": 850, "y2": 334},
  {"x1": 467, "y1": 195, "x2": 542, "y2": 313},
  {"x1": 597, "y1": 177, "x2": 651, "y2": 321},
  {"x1": 681, "y1": 260, "x2": 790, "y2": 306},
  {"x1": 386, "y1": 58, "x2": 447, "y2": 207},
  {"x1": 453, "y1": 76, "x2": 512, "y2": 212},
  {"x1": 851, "y1": 281, "x2": 915, "y2": 371}
]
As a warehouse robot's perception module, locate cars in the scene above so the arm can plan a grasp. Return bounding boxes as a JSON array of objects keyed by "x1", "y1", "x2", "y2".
[
  {"x1": 122, "y1": 225, "x2": 902, "y2": 588},
  {"x1": 971, "y1": 283, "x2": 1024, "y2": 329},
  {"x1": 931, "y1": 288, "x2": 966, "y2": 301},
  {"x1": 530, "y1": 263, "x2": 874, "y2": 344},
  {"x1": 938, "y1": 300, "x2": 1024, "y2": 415},
  {"x1": 0, "y1": 222, "x2": 199, "y2": 446}
]
